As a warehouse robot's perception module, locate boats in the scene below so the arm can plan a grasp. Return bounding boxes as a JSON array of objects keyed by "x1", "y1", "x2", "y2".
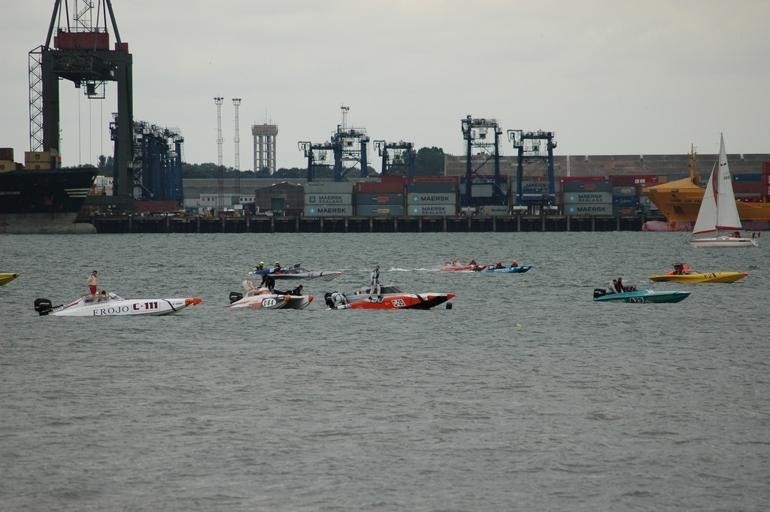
[
  {"x1": 440, "y1": 258, "x2": 532, "y2": 274},
  {"x1": 0, "y1": 272, "x2": 20, "y2": 286},
  {"x1": 34, "y1": 292, "x2": 201, "y2": 317},
  {"x1": 325, "y1": 284, "x2": 455, "y2": 310},
  {"x1": 229, "y1": 279, "x2": 313, "y2": 311},
  {"x1": 248, "y1": 264, "x2": 342, "y2": 282},
  {"x1": 593, "y1": 289, "x2": 691, "y2": 304},
  {"x1": 648, "y1": 271, "x2": 748, "y2": 284}
]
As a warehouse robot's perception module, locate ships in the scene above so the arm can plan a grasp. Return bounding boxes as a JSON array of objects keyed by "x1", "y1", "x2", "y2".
[{"x1": 641, "y1": 142, "x2": 770, "y2": 230}]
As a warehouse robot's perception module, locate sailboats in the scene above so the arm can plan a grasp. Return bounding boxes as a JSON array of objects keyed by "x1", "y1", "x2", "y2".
[{"x1": 688, "y1": 130, "x2": 758, "y2": 248}]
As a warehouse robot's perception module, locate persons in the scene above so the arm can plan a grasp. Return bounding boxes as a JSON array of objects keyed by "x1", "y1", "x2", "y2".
[
  {"x1": 291, "y1": 285, "x2": 303, "y2": 296},
  {"x1": 605, "y1": 279, "x2": 619, "y2": 295},
  {"x1": 88, "y1": 270, "x2": 97, "y2": 296},
  {"x1": 666, "y1": 263, "x2": 689, "y2": 275},
  {"x1": 449, "y1": 258, "x2": 478, "y2": 266},
  {"x1": 368, "y1": 264, "x2": 383, "y2": 300},
  {"x1": 496, "y1": 261, "x2": 518, "y2": 269},
  {"x1": 259, "y1": 275, "x2": 275, "y2": 293},
  {"x1": 274, "y1": 262, "x2": 282, "y2": 274},
  {"x1": 324, "y1": 292, "x2": 349, "y2": 308},
  {"x1": 97, "y1": 290, "x2": 107, "y2": 304},
  {"x1": 258, "y1": 262, "x2": 264, "y2": 272},
  {"x1": 615, "y1": 276, "x2": 625, "y2": 294}
]
